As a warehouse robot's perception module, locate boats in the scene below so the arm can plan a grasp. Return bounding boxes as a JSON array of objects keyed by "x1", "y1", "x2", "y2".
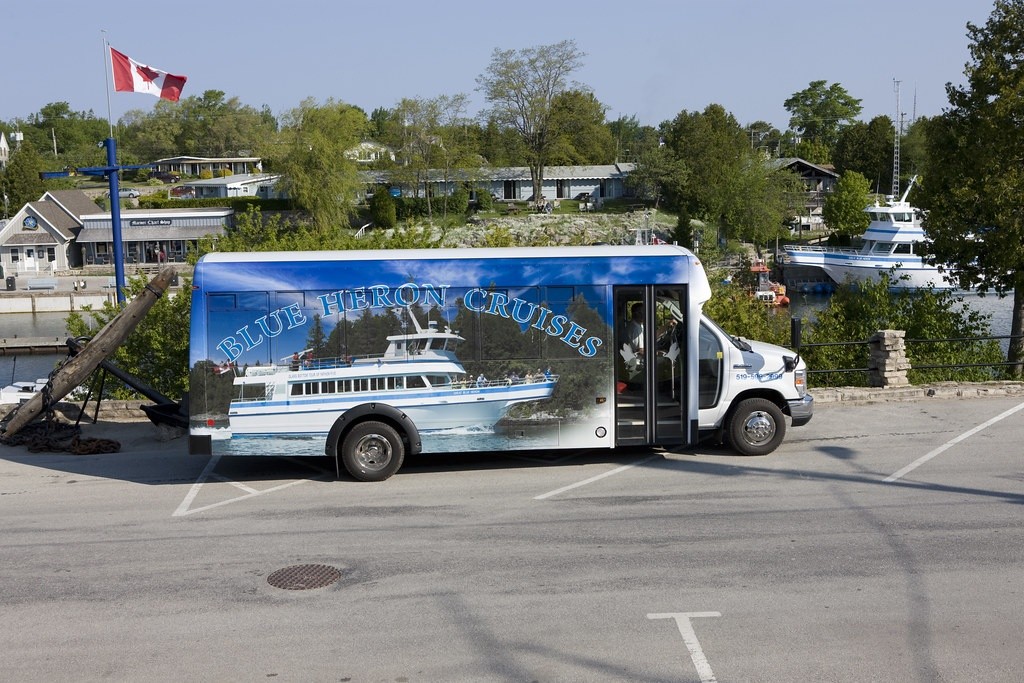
[
  {"x1": 776, "y1": 174, "x2": 1014, "y2": 297},
  {"x1": 227, "y1": 305, "x2": 561, "y2": 434}
]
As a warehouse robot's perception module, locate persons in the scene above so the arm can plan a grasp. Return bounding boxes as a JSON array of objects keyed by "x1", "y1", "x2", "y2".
[
  {"x1": 503, "y1": 366, "x2": 552, "y2": 386},
  {"x1": 293, "y1": 352, "x2": 317, "y2": 370},
  {"x1": 448, "y1": 374, "x2": 487, "y2": 390},
  {"x1": 626, "y1": 303, "x2": 676, "y2": 383}
]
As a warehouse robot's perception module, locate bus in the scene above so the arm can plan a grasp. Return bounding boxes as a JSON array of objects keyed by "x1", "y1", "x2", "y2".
[{"x1": 187, "y1": 245, "x2": 814, "y2": 484}]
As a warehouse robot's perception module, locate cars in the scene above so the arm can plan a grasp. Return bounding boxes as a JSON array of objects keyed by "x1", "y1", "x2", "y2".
[
  {"x1": 148, "y1": 171, "x2": 181, "y2": 183},
  {"x1": 105, "y1": 187, "x2": 140, "y2": 198},
  {"x1": 388, "y1": 186, "x2": 401, "y2": 197},
  {"x1": 173, "y1": 186, "x2": 195, "y2": 196}
]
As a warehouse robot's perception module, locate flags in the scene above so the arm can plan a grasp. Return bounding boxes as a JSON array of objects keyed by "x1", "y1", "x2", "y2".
[
  {"x1": 212, "y1": 359, "x2": 234, "y2": 374},
  {"x1": 109, "y1": 47, "x2": 187, "y2": 102}
]
